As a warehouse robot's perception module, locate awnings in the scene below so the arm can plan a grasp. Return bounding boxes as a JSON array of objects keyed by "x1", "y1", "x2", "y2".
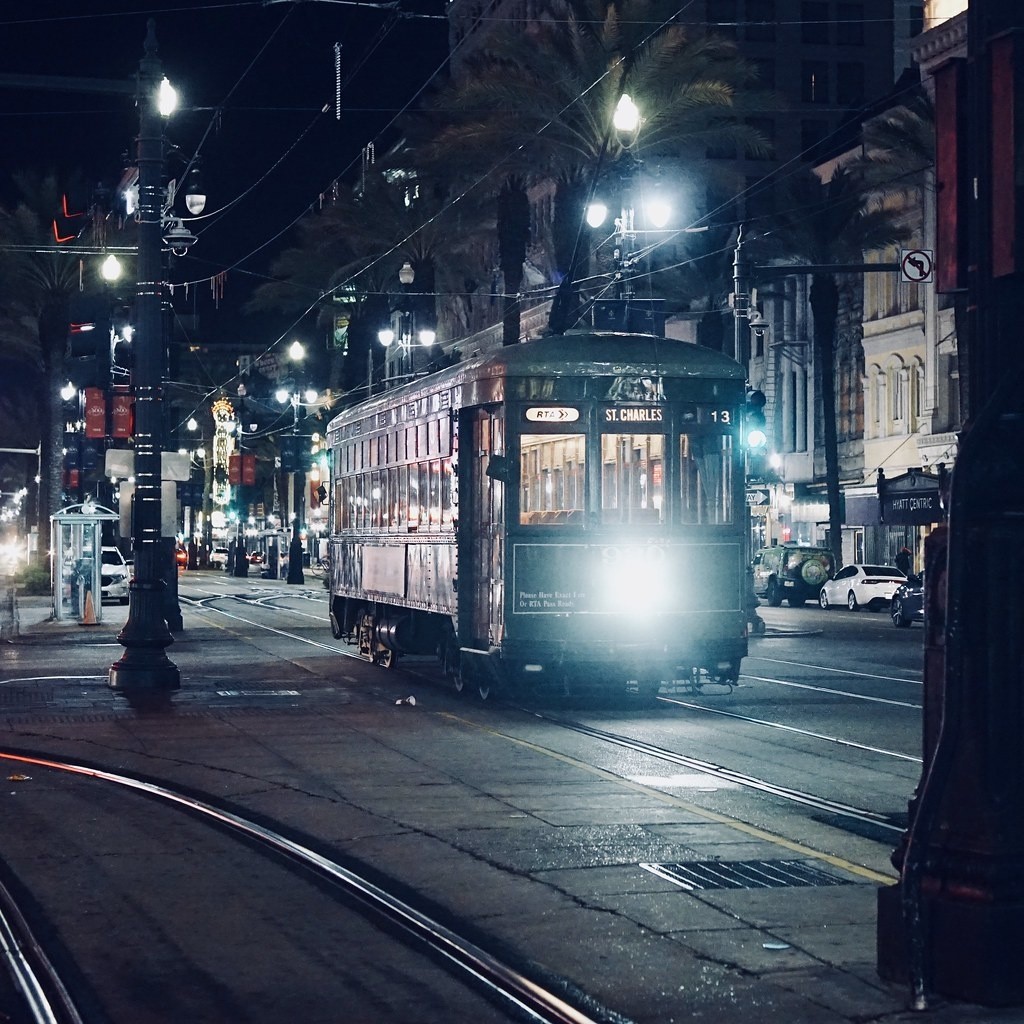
[{"x1": 791, "y1": 490, "x2": 944, "y2": 527}]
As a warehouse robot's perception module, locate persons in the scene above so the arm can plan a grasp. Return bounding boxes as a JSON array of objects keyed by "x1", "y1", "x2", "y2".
[{"x1": 894, "y1": 546, "x2": 912, "y2": 575}]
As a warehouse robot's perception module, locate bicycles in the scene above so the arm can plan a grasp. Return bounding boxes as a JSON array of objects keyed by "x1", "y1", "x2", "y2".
[{"x1": 311, "y1": 557, "x2": 329, "y2": 575}]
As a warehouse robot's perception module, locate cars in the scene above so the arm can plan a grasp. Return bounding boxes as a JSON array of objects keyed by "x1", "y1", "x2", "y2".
[
  {"x1": 818, "y1": 563, "x2": 910, "y2": 611},
  {"x1": 891, "y1": 570, "x2": 925, "y2": 628},
  {"x1": 62, "y1": 547, "x2": 134, "y2": 607}
]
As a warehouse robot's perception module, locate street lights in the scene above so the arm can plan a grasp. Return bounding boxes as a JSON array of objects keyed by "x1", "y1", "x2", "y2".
[
  {"x1": 584, "y1": 86, "x2": 687, "y2": 333},
  {"x1": 122, "y1": 73, "x2": 214, "y2": 632},
  {"x1": 275, "y1": 340, "x2": 318, "y2": 584},
  {"x1": 94, "y1": 255, "x2": 134, "y2": 546},
  {"x1": 377, "y1": 259, "x2": 437, "y2": 385},
  {"x1": 227, "y1": 382, "x2": 260, "y2": 576},
  {"x1": 178, "y1": 418, "x2": 207, "y2": 569}
]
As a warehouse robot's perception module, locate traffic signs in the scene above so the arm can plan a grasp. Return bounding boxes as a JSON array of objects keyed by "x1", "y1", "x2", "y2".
[{"x1": 743, "y1": 488, "x2": 770, "y2": 506}]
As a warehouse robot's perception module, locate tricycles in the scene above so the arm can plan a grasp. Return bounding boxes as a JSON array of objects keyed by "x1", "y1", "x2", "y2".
[{"x1": 175, "y1": 548, "x2": 186, "y2": 570}]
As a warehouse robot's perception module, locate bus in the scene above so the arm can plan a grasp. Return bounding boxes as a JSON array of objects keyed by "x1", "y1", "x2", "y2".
[{"x1": 325, "y1": 328, "x2": 750, "y2": 707}]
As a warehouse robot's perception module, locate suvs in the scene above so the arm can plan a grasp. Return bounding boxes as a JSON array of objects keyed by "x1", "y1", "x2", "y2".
[
  {"x1": 751, "y1": 542, "x2": 837, "y2": 608},
  {"x1": 251, "y1": 552, "x2": 266, "y2": 564},
  {"x1": 209, "y1": 547, "x2": 229, "y2": 564}
]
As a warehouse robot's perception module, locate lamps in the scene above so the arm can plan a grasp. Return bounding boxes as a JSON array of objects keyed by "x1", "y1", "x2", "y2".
[{"x1": 185, "y1": 165, "x2": 207, "y2": 215}]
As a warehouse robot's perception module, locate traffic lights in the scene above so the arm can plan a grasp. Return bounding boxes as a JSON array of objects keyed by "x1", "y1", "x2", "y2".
[{"x1": 745, "y1": 389, "x2": 767, "y2": 457}]
